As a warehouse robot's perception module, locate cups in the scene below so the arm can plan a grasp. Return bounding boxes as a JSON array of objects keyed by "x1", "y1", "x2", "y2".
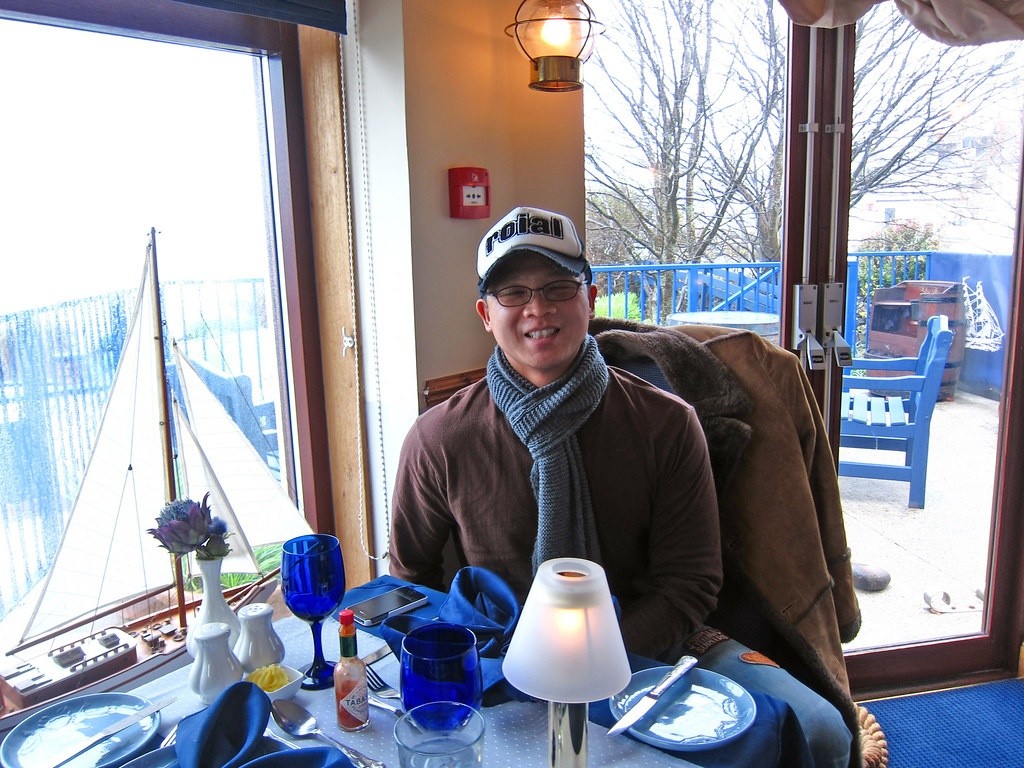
[
  {"x1": 398, "y1": 623, "x2": 484, "y2": 738},
  {"x1": 393, "y1": 700, "x2": 486, "y2": 768}
]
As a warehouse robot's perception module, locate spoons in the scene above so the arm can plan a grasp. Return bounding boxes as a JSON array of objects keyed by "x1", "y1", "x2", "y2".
[{"x1": 271, "y1": 699, "x2": 386, "y2": 768}]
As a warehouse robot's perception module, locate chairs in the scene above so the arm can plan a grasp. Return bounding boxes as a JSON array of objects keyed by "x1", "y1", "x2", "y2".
[{"x1": 838, "y1": 314, "x2": 953, "y2": 509}]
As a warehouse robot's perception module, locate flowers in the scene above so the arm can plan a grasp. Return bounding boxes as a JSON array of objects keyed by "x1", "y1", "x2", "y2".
[{"x1": 144, "y1": 490, "x2": 236, "y2": 566}]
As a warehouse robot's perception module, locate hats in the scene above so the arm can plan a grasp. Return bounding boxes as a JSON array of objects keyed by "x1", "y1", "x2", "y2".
[{"x1": 476, "y1": 206, "x2": 593, "y2": 299}]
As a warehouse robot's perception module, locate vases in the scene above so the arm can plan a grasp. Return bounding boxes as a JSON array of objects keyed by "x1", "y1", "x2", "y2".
[{"x1": 186, "y1": 558, "x2": 241, "y2": 660}]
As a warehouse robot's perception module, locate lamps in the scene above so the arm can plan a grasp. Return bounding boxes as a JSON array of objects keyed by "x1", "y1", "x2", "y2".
[
  {"x1": 501, "y1": 557, "x2": 632, "y2": 768},
  {"x1": 503, "y1": 0, "x2": 607, "y2": 93}
]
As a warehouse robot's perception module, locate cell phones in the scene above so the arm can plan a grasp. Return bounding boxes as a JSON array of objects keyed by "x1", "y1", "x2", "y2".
[{"x1": 344, "y1": 585, "x2": 429, "y2": 627}]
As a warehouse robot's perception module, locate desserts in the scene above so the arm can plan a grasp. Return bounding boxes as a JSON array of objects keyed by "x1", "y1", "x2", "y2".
[{"x1": 243, "y1": 664, "x2": 288, "y2": 691}]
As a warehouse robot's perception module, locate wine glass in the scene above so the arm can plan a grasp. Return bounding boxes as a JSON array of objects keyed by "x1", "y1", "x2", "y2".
[{"x1": 280, "y1": 532, "x2": 346, "y2": 691}]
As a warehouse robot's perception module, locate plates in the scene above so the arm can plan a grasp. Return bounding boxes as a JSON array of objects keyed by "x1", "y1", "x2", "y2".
[
  {"x1": 0, "y1": 693, "x2": 161, "y2": 768},
  {"x1": 609, "y1": 666, "x2": 757, "y2": 752}
]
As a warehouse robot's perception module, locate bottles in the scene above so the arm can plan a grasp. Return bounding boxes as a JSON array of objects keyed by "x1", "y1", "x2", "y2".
[
  {"x1": 190, "y1": 622, "x2": 244, "y2": 705},
  {"x1": 333, "y1": 608, "x2": 371, "y2": 731},
  {"x1": 233, "y1": 602, "x2": 286, "y2": 673}
]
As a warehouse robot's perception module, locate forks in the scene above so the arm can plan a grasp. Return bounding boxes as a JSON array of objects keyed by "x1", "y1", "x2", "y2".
[{"x1": 363, "y1": 662, "x2": 401, "y2": 699}]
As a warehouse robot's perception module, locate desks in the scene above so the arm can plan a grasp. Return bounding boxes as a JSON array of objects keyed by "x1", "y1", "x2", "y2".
[{"x1": 127, "y1": 574, "x2": 818, "y2": 768}]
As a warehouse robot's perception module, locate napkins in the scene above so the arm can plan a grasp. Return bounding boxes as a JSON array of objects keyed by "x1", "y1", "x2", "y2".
[
  {"x1": 380, "y1": 564, "x2": 620, "y2": 708},
  {"x1": 175, "y1": 681, "x2": 355, "y2": 768}
]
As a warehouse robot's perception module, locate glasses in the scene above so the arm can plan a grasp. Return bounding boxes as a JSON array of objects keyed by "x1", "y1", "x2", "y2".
[{"x1": 483, "y1": 279, "x2": 589, "y2": 307}]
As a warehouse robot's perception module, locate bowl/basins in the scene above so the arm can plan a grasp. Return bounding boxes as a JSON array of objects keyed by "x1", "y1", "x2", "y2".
[{"x1": 242, "y1": 663, "x2": 305, "y2": 705}]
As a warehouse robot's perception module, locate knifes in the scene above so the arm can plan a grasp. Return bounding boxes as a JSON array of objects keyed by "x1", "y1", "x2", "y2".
[
  {"x1": 604, "y1": 655, "x2": 700, "y2": 736},
  {"x1": 360, "y1": 643, "x2": 392, "y2": 665},
  {"x1": 46, "y1": 695, "x2": 180, "y2": 768}
]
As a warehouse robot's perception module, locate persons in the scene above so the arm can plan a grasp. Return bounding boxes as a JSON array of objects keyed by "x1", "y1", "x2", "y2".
[{"x1": 388, "y1": 207, "x2": 723, "y2": 664}]
